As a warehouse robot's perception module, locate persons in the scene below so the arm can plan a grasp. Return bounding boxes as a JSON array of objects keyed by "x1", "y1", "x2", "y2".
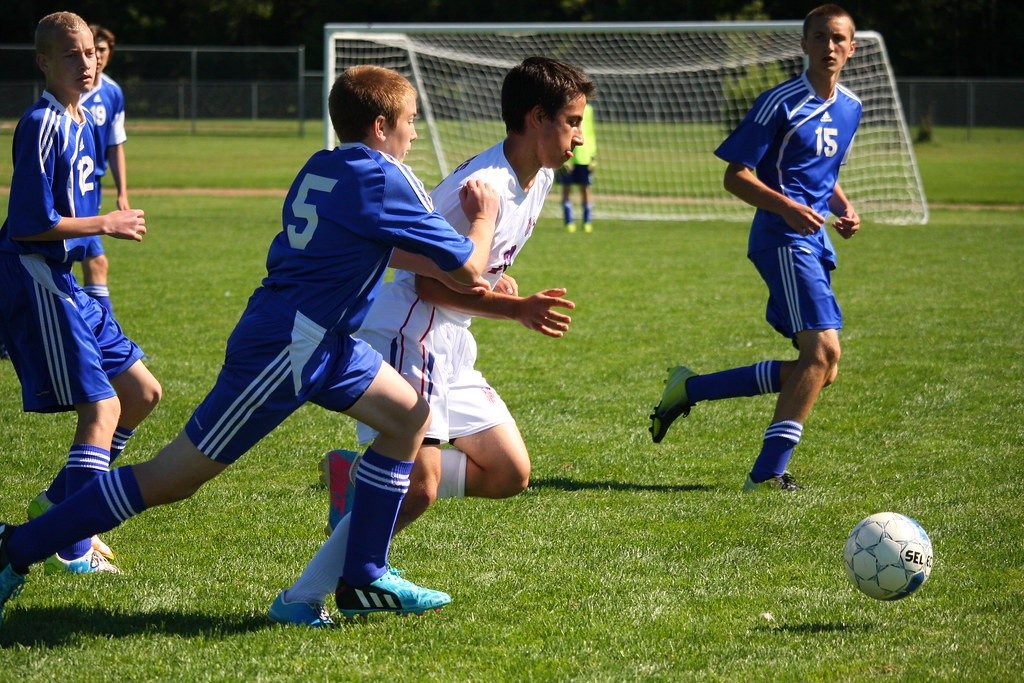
[
  {"x1": 318, "y1": 56, "x2": 596, "y2": 538},
  {"x1": 0, "y1": 11, "x2": 162, "y2": 575},
  {"x1": 0, "y1": 64, "x2": 499, "y2": 615},
  {"x1": 551, "y1": 103, "x2": 597, "y2": 234},
  {"x1": 648, "y1": 5, "x2": 863, "y2": 492}
]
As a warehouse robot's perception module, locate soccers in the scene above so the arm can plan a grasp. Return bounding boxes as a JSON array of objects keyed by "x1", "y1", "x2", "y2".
[{"x1": 840, "y1": 509, "x2": 936, "y2": 604}]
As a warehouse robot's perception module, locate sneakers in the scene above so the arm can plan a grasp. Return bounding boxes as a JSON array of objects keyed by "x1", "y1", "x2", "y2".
[
  {"x1": 648, "y1": 366, "x2": 701, "y2": 445},
  {"x1": 565, "y1": 220, "x2": 593, "y2": 235},
  {"x1": 740, "y1": 470, "x2": 810, "y2": 494},
  {"x1": 335, "y1": 562, "x2": 454, "y2": 621},
  {"x1": 0, "y1": 521, "x2": 25, "y2": 620},
  {"x1": 318, "y1": 450, "x2": 364, "y2": 537},
  {"x1": 265, "y1": 592, "x2": 351, "y2": 630},
  {"x1": 25, "y1": 490, "x2": 126, "y2": 576}
]
